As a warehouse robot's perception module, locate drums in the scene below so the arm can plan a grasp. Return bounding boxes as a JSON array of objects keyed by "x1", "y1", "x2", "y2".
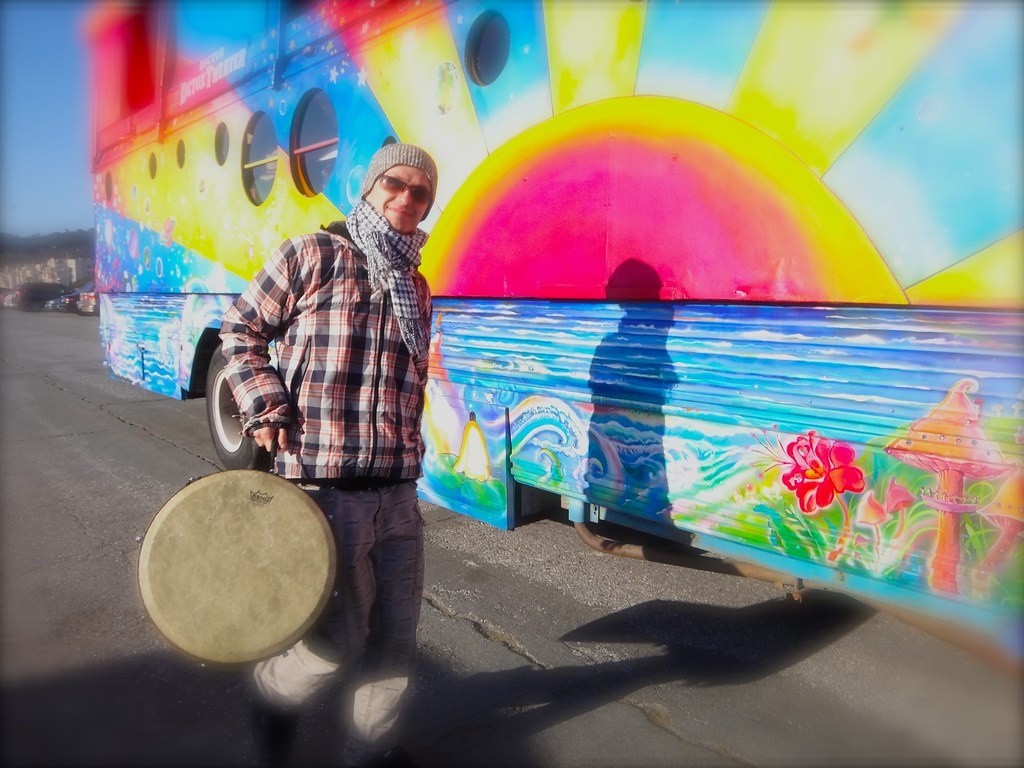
[{"x1": 136, "y1": 465, "x2": 341, "y2": 668}]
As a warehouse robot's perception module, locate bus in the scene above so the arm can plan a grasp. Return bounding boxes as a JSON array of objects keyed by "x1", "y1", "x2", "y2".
[{"x1": 91, "y1": 5, "x2": 1022, "y2": 644}]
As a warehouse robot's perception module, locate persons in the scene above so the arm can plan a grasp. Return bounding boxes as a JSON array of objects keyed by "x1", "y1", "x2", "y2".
[{"x1": 220, "y1": 144, "x2": 438, "y2": 768}]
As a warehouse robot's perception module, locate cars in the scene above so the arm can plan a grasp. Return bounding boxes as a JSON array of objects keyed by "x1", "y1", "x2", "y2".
[
  {"x1": 0, "y1": 287, "x2": 18, "y2": 310},
  {"x1": 57, "y1": 290, "x2": 81, "y2": 316},
  {"x1": 46, "y1": 297, "x2": 64, "y2": 313}
]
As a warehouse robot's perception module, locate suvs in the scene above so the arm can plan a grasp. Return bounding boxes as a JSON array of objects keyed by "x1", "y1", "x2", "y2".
[
  {"x1": 80, "y1": 278, "x2": 99, "y2": 315},
  {"x1": 11, "y1": 283, "x2": 75, "y2": 310}
]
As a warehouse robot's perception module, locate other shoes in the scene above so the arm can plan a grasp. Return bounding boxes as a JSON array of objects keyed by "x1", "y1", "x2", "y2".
[
  {"x1": 345, "y1": 744, "x2": 418, "y2": 768},
  {"x1": 247, "y1": 697, "x2": 298, "y2": 768}
]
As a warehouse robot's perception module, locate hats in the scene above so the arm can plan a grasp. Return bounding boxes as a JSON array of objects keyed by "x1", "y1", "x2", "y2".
[{"x1": 360, "y1": 143, "x2": 438, "y2": 222}]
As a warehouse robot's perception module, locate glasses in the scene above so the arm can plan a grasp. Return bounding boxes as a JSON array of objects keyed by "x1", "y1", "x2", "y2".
[{"x1": 380, "y1": 175, "x2": 432, "y2": 205}]
